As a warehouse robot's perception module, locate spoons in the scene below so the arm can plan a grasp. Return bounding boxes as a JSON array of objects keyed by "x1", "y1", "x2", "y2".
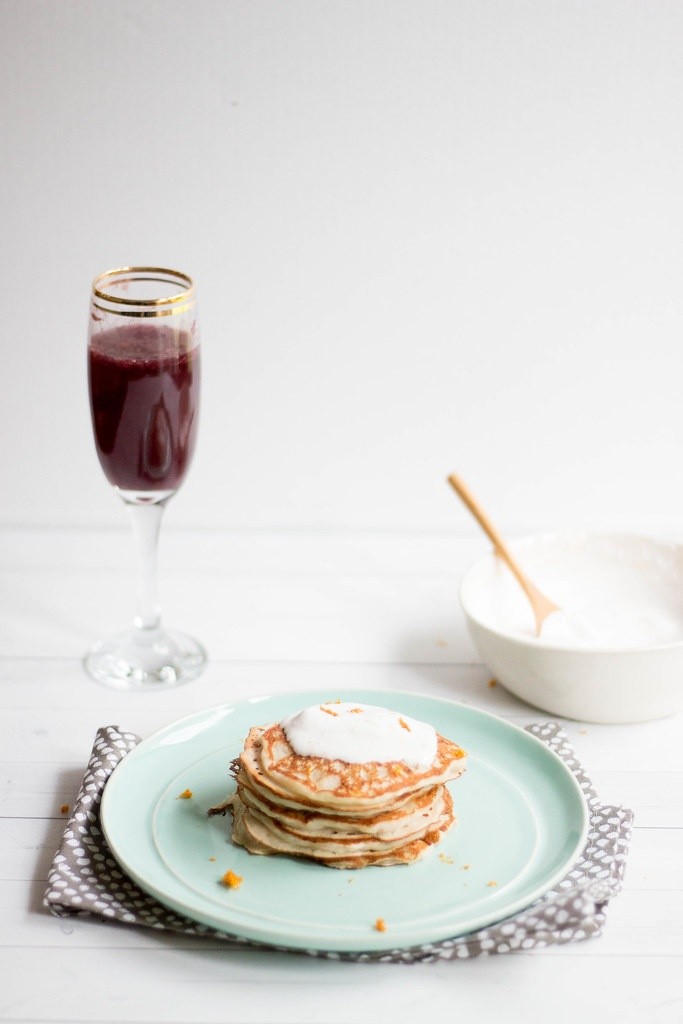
[{"x1": 447, "y1": 475, "x2": 561, "y2": 637}]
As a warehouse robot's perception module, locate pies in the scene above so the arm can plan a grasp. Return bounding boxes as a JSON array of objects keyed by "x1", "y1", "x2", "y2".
[{"x1": 225, "y1": 718, "x2": 470, "y2": 870}]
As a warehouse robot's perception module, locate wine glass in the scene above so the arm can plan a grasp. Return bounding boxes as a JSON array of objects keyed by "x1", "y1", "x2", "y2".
[{"x1": 84, "y1": 267, "x2": 204, "y2": 691}]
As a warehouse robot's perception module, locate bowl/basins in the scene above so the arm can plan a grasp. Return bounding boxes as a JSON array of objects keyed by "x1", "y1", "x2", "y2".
[{"x1": 459, "y1": 528, "x2": 683, "y2": 723}]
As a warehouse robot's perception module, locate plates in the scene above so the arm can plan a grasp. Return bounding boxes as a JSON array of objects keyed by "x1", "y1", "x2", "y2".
[{"x1": 103, "y1": 688, "x2": 590, "y2": 951}]
